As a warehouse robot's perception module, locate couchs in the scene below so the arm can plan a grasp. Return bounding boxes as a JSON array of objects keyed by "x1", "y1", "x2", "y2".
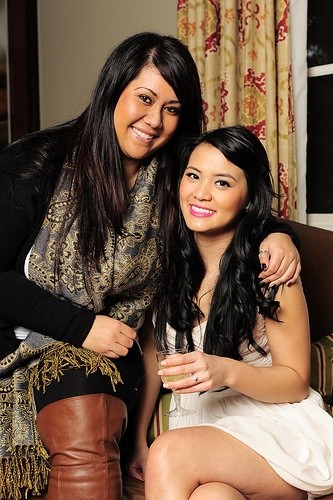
[{"x1": 270, "y1": 216, "x2": 333, "y2": 418}]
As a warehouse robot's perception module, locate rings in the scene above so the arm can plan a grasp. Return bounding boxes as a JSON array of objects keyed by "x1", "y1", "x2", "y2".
[
  {"x1": 259, "y1": 250, "x2": 270, "y2": 254},
  {"x1": 192, "y1": 374, "x2": 198, "y2": 384}
]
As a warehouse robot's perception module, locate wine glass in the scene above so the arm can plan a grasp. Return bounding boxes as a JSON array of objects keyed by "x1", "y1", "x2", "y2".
[{"x1": 155, "y1": 349, "x2": 196, "y2": 417}]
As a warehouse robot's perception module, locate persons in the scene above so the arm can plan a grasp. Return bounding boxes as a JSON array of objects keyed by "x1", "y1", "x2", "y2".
[
  {"x1": 0, "y1": 31, "x2": 302, "y2": 499},
  {"x1": 125, "y1": 125, "x2": 333, "y2": 500}
]
